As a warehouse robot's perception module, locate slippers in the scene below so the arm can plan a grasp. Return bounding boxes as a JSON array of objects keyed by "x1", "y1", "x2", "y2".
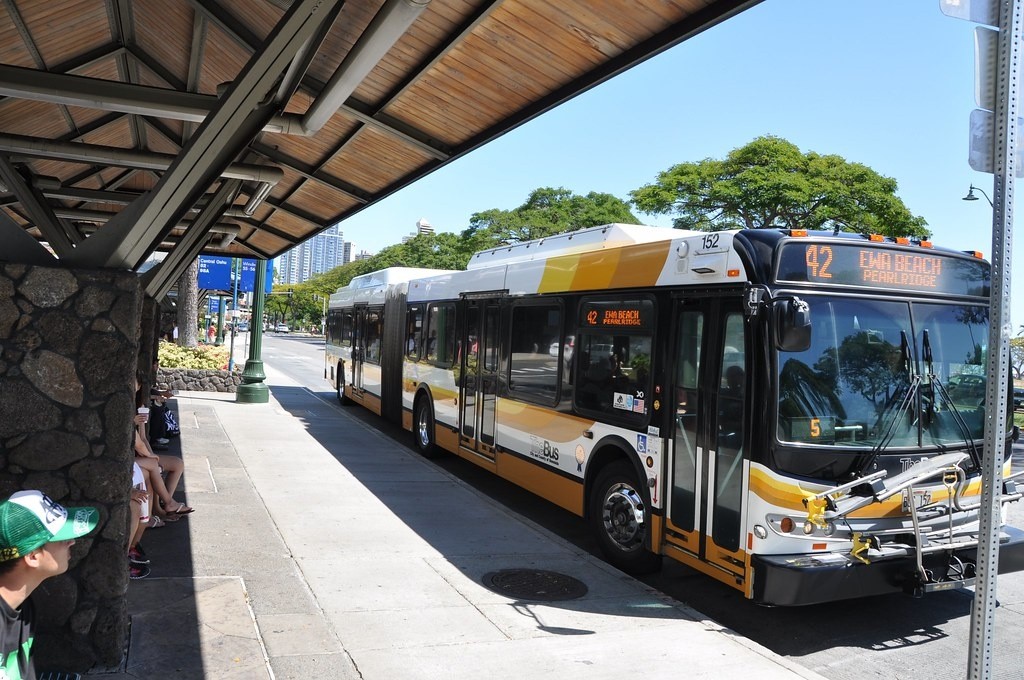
[
  {"x1": 145, "y1": 516, "x2": 165, "y2": 530},
  {"x1": 166, "y1": 502, "x2": 195, "y2": 515}
]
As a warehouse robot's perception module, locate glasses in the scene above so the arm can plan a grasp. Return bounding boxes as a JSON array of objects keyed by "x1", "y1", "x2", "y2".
[{"x1": 152, "y1": 364, "x2": 158, "y2": 367}]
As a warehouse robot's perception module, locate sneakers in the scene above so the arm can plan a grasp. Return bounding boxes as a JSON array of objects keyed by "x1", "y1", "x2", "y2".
[
  {"x1": 129, "y1": 562, "x2": 151, "y2": 580},
  {"x1": 128, "y1": 541, "x2": 150, "y2": 564}
]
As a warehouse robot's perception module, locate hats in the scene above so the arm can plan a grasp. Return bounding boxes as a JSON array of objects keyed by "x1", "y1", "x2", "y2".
[{"x1": 0, "y1": 490, "x2": 99, "y2": 561}]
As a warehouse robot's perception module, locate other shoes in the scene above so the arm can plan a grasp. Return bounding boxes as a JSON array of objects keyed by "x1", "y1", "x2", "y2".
[
  {"x1": 157, "y1": 438, "x2": 169, "y2": 444},
  {"x1": 156, "y1": 511, "x2": 182, "y2": 521}
]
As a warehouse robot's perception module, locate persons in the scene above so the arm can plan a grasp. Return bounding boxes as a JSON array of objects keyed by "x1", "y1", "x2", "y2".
[
  {"x1": 406, "y1": 321, "x2": 415, "y2": 357},
  {"x1": 861, "y1": 336, "x2": 912, "y2": 409},
  {"x1": 426, "y1": 329, "x2": 438, "y2": 355},
  {"x1": 130, "y1": 355, "x2": 193, "y2": 579},
  {"x1": 608, "y1": 351, "x2": 630, "y2": 392},
  {"x1": 171, "y1": 322, "x2": 179, "y2": 343},
  {"x1": 570, "y1": 351, "x2": 610, "y2": 411},
  {"x1": 724, "y1": 365, "x2": 745, "y2": 390},
  {"x1": 0, "y1": 488, "x2": 77, "y2": 679},
  {"x1": 208, "y1": 322, "x2": 217, "y2": 345}
]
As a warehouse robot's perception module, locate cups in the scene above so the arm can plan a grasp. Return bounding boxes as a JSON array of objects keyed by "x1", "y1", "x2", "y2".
[
  {"x1": 138, "y1": 408, "x2": 149, "y2": 423},
  {"x1": 139, "y1": 495, "x2": 149, "y2": 523}
]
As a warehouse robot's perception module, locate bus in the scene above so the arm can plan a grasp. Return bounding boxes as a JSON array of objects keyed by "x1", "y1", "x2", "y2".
[{"x1": 324, "y1": 222, "x2": 1024, "y2": 608}]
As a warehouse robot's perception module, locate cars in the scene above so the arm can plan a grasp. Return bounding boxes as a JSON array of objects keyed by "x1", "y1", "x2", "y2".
[
  {"x1": 237, "y1": 320, "x2": 267, "y2": 333},
  {"x1": 548, "y1": 333, "x2": 748, "y2": 377},
  {"x1": 275, "y1": 324, "x2": 289, "y2": 334}
]
